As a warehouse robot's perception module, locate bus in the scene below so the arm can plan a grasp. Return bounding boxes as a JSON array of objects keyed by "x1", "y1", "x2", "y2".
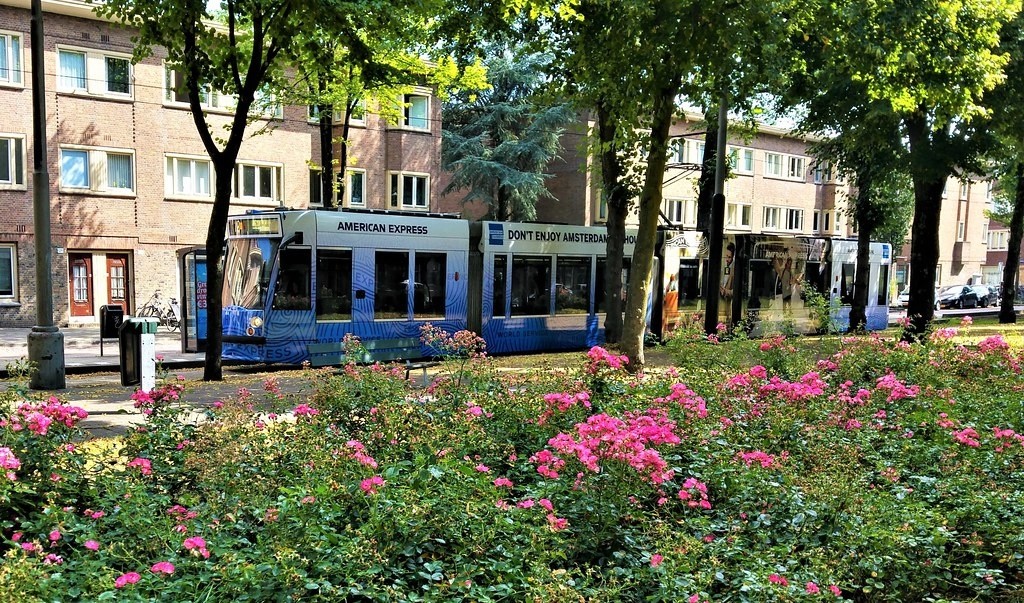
[{"x1": 220, "y1": 165, "x2": 891, "y2": 367}]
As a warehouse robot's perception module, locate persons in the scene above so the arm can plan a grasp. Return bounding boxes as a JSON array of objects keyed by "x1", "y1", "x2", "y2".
[
  {"x1": 664, "y1": 274, "x2": 676, "y2": 301},
  {"x1": 771, "y1": 253, "x2": 795, "y2": 317},
  {"x1": 719, "y1": 241, "x2": 736, "y2": 319}
]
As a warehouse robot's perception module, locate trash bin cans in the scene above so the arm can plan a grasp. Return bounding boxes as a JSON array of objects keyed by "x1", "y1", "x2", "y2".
[
  {"x1": 100, "y1": 304, "x2": 124, "y2": 338},
  {"x1": 118, "y1": 317, "x2": 160, "y2": 387}
]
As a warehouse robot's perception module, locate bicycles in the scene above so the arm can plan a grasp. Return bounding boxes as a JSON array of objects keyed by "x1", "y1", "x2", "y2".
[{"x1": 137, "y1": 289, "x2": 181, "y2": 332}]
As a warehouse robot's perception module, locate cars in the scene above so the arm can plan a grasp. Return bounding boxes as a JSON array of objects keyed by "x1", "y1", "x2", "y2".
[
  {"x1": 897, "y1": 284, "x2": 941, "y2": 311},
  {"x1": 970, "y1": 282, "x2": 1024, "y2": 308},
  {"x1": 939, "y1": 284, "x2": 978, "y2": 309}
]
